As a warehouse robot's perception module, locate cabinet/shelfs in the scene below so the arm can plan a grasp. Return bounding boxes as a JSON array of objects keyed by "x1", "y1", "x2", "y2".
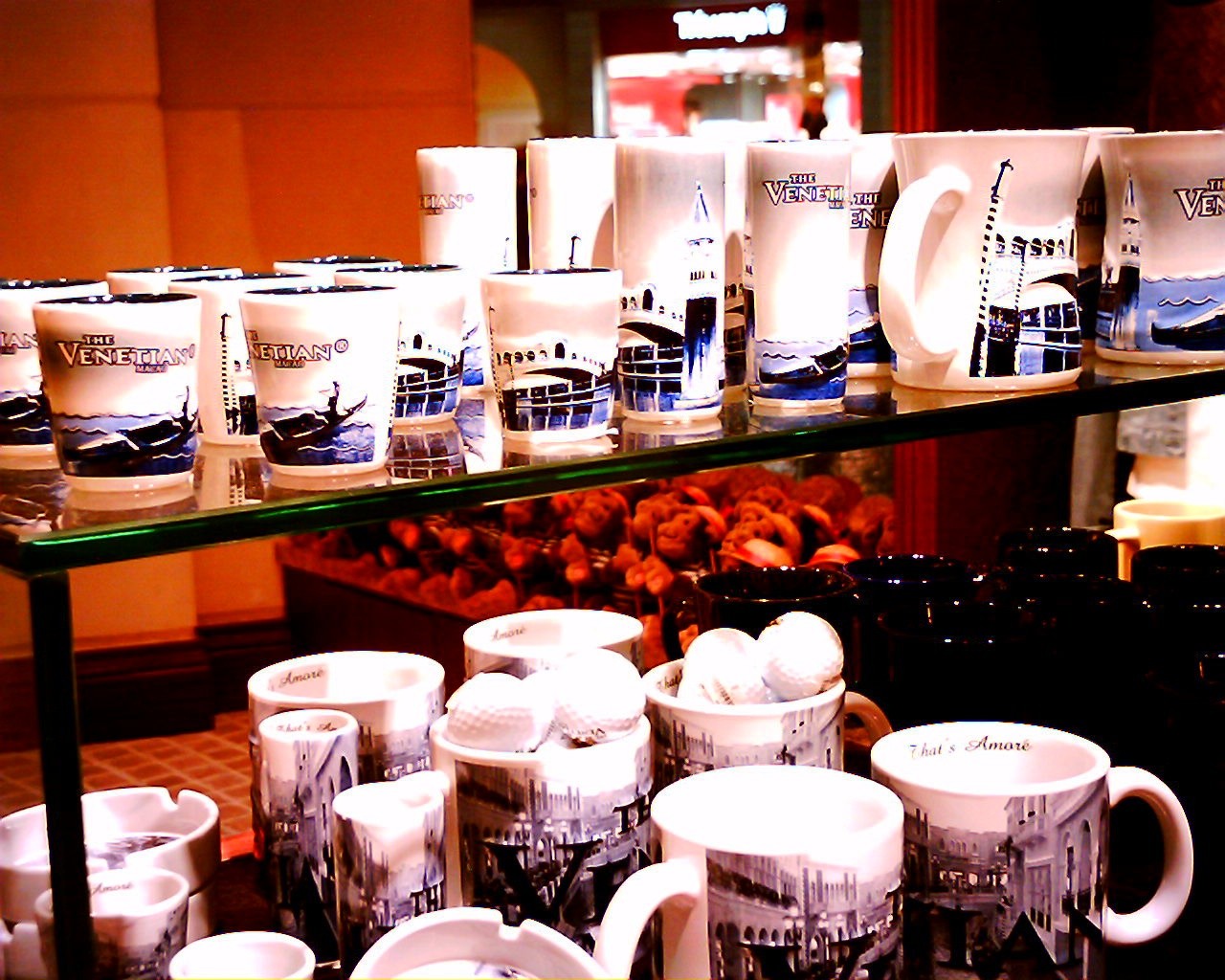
[{"x1": 0, "y1": 363, "x2": 1225, "y2": 980}]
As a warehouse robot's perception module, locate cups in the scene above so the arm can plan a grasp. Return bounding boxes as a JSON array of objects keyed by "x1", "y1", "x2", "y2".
[
  {"x1": 870, "y1": 721, "x2": 1195, "y2": 980},
  {"x1": 847, "y1": 133, "x2": 907, "y2": 379},
  {"x1": 273, "y1": 255, "x2": 402, "y2": 282},
  {"x1": 592, "y1": 766, "x2": 904, "y2": 980},
  {"x1": 168, "y1": 272, "x2": 316, "y2": 445},
  {"x1": 662, "y1": 498, "x2": 1225, "y2": 980},
  {"x1": 1, "y1": 279, "x2": 110, "y2": 470},
  {"x1": 249, "y1": 609, "x2": 895, "y2": 970},
  {"x1": 480, "y1": 267, "x2": 623, "y2": 444},
  {"x1": 1074, "y1": 127, "x2": 1225, "y2": 365},
  {"x1": 878, "y1": 129, "x2": 1090, "y2": 393},
  {"x1": 32, "y1": 291, "x2": 201, "y2": 491},
  {"x1": 106, "y1": 266, "x2": 244, "y2": 296},
  {"x1": 334, "y1": 264, "x2": 467, "y2": 425},
  {"x1": 238, "y1": 284, "x2": 400, "y2": 478}
]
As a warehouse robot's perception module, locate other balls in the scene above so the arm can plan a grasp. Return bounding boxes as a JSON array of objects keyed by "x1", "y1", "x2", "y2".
[
  {"x1": 441, "y1": 646, "x2": 649, "y2": 755},
  {"x1": 674, "y1": 608, "x2": 845, "y2": 710}
]
